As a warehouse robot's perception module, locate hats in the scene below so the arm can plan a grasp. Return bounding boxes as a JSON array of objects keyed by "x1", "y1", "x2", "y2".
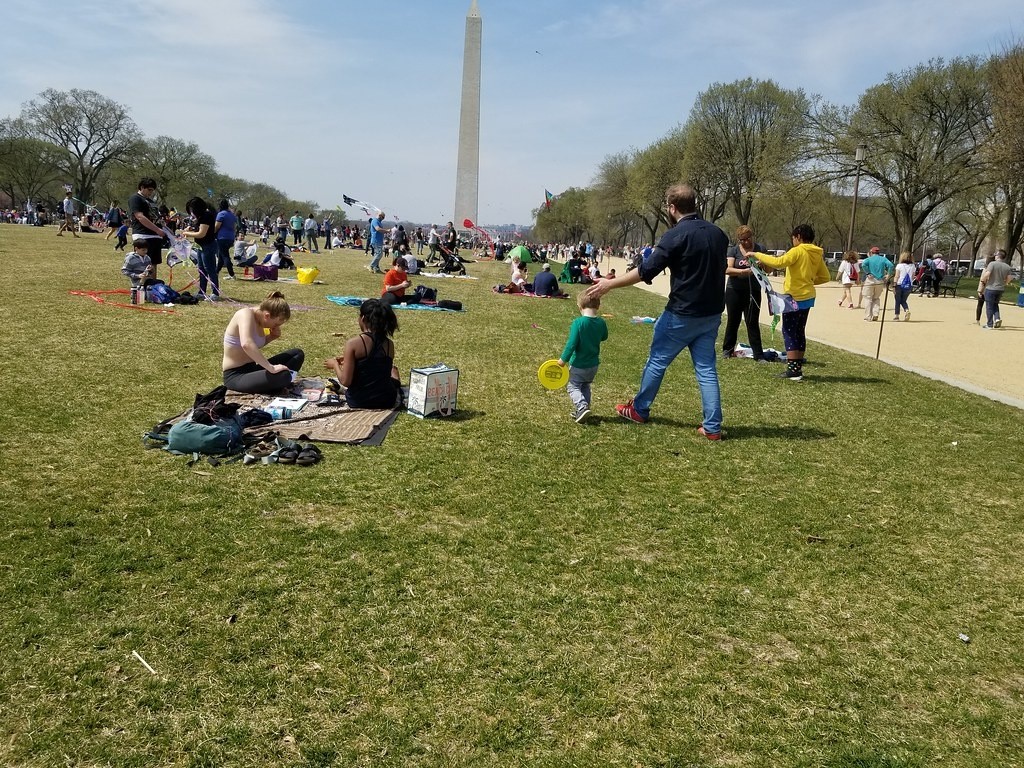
[
  {"x1": 871, "y1": 247, "x2": 880, "y2": 253},
  {"x1": 396, "y1": 257, "x2": 409, "y2": 270}
]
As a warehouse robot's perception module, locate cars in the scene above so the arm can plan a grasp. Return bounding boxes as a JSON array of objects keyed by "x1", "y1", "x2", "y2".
[
  {"x1": 857, "y1": 253, "x2": 870, "y2": 270},
  {"x1": 824, "y1": 251, "x2": 843, "y2": 263},
  {"x1": 1010, "y1": 272, "x2": 1020, "y2": 281}
]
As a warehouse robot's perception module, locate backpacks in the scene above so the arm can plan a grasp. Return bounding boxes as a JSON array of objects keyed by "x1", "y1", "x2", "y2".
[
  {"x1": 924, "y1": 265, "x2": 933, "y2": 274},
  {"x1": 146, "y1": 283, "x2": 178, "y2": 303},
  {"x1": 844, "y1": 260, "x2": 858, "y2": 281},
  {"x1": 901, "y1": 264, "x2": 912, "y2": 289}
]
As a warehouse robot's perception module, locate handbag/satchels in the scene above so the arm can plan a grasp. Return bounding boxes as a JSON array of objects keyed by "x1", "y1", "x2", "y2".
[
  {"x1": 254, "y1": 264, "x2": 278, "y2": 281},
  {"x1": 167, "y1": 416, "x2": 242, "y2": 451},
  {"x1": 414, "y1": 285, "x2": 439, "y2": 307},
  {"x1": 408, "y1": 364, "x2": 460, "y2": 420},
  {"x1": 297, "y1": 265, "x2": 320, "y2": 284}
]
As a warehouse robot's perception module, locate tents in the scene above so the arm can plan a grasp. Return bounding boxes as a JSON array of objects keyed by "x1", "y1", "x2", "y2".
[{"x1": 503, "y1": 245, "x2": 533, "y2": 263}]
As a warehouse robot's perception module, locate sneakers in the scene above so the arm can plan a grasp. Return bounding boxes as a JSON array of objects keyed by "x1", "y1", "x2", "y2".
[
  {"x1": 616, "y1": 400, "x2": 645, "y2": 425},
  {"x1": 223, "y1": 273, "x2": 236, "y2": 281},
  {"x1": 193, "y1": 293, "x2": 203, "y2": 299},
  {"x1": 697, "y1": 427, "x2": 722, "y2": 440},
  {"x1": 571, "y1": 406, "x2": 591, "y2": 422},
  {"x1": 779, "y1": 369, "x2": 802, "y2": 381},
  {"x1": 204, "y1": 293, "x2": 219, "y2": 301}
]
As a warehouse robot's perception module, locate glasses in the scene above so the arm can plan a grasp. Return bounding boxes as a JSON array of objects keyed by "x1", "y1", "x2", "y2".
[{"x1": 740, "y1": 235, "x2": 753, "y2": 243}]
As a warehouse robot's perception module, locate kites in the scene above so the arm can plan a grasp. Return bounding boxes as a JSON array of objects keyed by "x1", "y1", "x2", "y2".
[
  {"x1": 463, "y1": 218, "x2": 495, "y2": 261},
  {"x1": 69, "y1": 225, "x2": 321, "y2": 316},
  {"x1": 62, "y1": 184, "x2": 100, "y2": 212},
  {"x1": 342, "y1": 194, "x2": 359, "y2": 206},
  {"x1": 748, "y1": 257, "x2": 798, "y2": 340},
  {"x1": 544, "y1": 189, "x2": 552, "y2": 210}
]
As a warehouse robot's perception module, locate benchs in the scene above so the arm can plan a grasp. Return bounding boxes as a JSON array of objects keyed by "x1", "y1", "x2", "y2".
[{"x1": 926, "y1": 275, "x2": 962, "y2": 297}]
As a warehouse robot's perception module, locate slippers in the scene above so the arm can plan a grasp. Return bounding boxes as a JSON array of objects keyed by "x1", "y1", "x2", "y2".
[{"x1": 249, "y1": 440, "x2": 323, "y2": 464}]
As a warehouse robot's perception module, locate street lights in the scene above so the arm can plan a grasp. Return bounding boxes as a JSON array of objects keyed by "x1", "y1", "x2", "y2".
[{"x1": 847, "y1": 143, "x2": 866, "y2": 251}]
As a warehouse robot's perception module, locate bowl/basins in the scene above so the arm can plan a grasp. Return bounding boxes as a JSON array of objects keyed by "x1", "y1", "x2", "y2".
[
  {"x1": 302, "y1": 380, "x2": 324, "y2": 389},
  {"x1": 300, "y1": 389, "x2": 321, "y2": 402}
]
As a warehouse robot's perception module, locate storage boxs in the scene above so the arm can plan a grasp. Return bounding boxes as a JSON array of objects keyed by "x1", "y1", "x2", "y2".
[
  {"x1": 254, "y1": 265, "x2": 278, "y2": 280},
  {"x1": 407, "y1": 363, "x2": 459, "y2": 421},
  {"x1": 414, "y1": 285, "x2": 438, "y2": 302}
]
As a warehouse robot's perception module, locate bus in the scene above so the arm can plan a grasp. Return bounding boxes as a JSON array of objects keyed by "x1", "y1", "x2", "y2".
[{"x1": 950, "y1": 260, "x2": 983, "y2": 277}]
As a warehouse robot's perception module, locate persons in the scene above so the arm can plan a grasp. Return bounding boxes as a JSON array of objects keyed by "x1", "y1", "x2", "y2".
[
  {"x1": 366, "y1": 213, "x2": 460, "y2": 307},
  {"x1": 836, "y1": 247, "x2": 945, "y2": 321},
  {"x1": 470, "y1": 234, "x2": 666, "y2": 297},
  {"x1": 587, "y1": 183, "x2": 728, "y2": 441},
  {"x1": 744, "y1": 224, "x2": 830, "y2": 381},
  {"x1": 324, "y1": 298, "x2": 400, "y2": 411},
  {"x1": 0, "y1": 178, "x2": 365, "y2": 303},
  {"x1": 723, "y1": 225, "x2": 774, "y2": 362},
  {"x1": 222, "y1": 291, "x2": 304, "y2": 393},
  {"x1": 557, "y1": 290, "x2": 608, "y2": 422},
  {"x1": 972, "y1": 250, "x2": 1011, "y2": 329}
]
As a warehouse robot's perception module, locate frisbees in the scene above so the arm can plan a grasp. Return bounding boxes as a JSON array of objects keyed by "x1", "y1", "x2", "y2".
[{"x1": 537, "y1": 359, "x2": 570, "y2": 389}]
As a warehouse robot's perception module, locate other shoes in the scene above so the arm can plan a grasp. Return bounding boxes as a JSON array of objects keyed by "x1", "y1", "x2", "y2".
[
  {"x1": 369, "y1": 264, "x2": 386, "y2": 275},
  {"x1": 994, "y1": 319, "x2": 1001, "y2": 328},
  {"x1": 984, "y1": 324, "x2": 992, "y2": 329},
  {"x1": 837, "y1": 300, "x2": 911, "y2": 321}
]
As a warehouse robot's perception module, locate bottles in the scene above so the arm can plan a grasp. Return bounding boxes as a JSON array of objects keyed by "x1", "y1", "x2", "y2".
[
  {"x1": 140, "y1": 268, "x2": 149, "y2": 285},
  {"x1": 244, "y1": 266, "x2": 249, "y2": 275},
  {"x1": 260, "y1": 406, "x2": 293, "y2": 418},
  {"x1": 129, "y1": 284, "x2": 153, "y2": 305}
]
